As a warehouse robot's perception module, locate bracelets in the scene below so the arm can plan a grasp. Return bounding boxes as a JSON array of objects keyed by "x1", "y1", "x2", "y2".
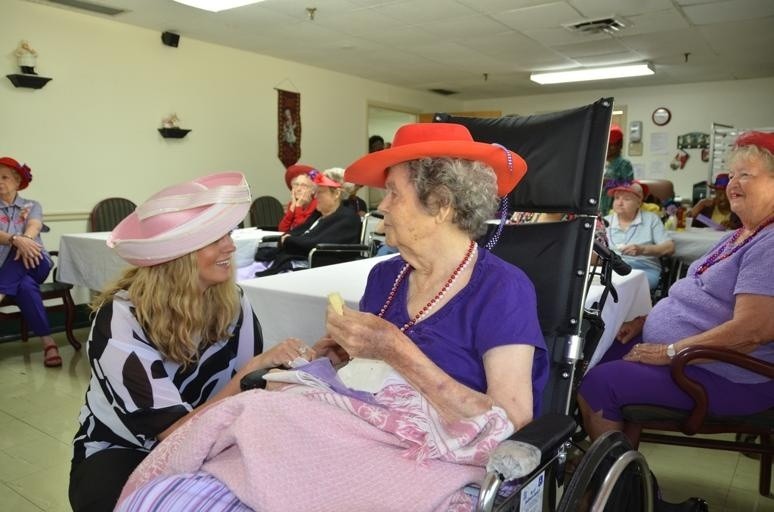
[{"x1": 8, "y1": 234, "x2": 17, "y2": 244}]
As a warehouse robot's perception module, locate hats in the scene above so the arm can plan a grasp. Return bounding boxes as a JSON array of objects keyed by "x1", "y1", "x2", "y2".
[
  {"x1": 284, "y1": 164, "x2": 320, "y2": 188},
  {"x1": 607, "y1": 186, "x2": 642, "y2": 199},
  {"x1": 105, "y1": 171, "x2": 252, "y2": 268},
  {"x1": 609, "y1": 125, "x2": 623, "y2": 143},
  {"x1": 708, "y1": 173, "x2": 730, "y2": 189},
  {"x1": 731, "y1": 130, "x2": 774, "y2": 156},
  {"x1": 0, "y1": 156, "x2": 32, "y2": 190},
  {"x1": 342, "y1": 122, "x2": 527, "y2": 198}
]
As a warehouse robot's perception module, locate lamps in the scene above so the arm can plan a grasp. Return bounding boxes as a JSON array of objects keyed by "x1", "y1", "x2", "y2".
[{"x1": 530, "y1": 60, "x2": 656, "y2": 85}]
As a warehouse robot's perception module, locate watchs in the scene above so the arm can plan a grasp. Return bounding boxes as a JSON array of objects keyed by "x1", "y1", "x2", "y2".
[{"x1": 666, "y1": 343, "x2": 675, "y2": 363}]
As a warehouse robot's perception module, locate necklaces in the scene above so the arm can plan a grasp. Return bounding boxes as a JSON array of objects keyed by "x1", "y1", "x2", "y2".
[
  {"x1": 693, "y1": 217, "x2": 774, "y2": 278},
  {"x1": 378, "y1": 239, "x2": 475, "y2": 337},
  {"x1": 295, "y1": 346, "x2": 308, "y2": 358}
]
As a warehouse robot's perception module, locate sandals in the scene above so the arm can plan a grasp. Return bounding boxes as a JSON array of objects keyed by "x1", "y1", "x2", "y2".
[{"x1": 43, "y1": 344, "x2": 63, "y2": 368}]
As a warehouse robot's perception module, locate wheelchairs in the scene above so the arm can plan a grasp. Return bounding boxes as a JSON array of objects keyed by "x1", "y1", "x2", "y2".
[
  {"x1": 241, "y1": 96, "x2": 660, "y2": 512},
  {"x1": 259, "y1": 210, "x2": 385, "y2": 271},
  {"x1": 649, "y1": 214, "x2": 683, "y2": 304}
]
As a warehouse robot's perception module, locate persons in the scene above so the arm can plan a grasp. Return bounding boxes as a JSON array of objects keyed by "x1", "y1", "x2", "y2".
[
  {"x1": 367, "y1": 134, "x2": 388, "y2": 209},
  {"x1": 274, "y1": 167, "x2": 363, "y2": 274},
  {"x1": 0, "y1": 156, "x2": 65, "y2": 368},
  {"x1": 278, "y1": 165, "x2": 317, "y2": 233},
  {"x1": 597, "y1": 126, "x2": 635, "y2": 218},
  {"x1": 591, "y1": 183, "x2": 676, "y2": 295},
  {"x1": 67, "y1": 170, "x2": 316, "y2": 511},
  {"x1": 685, "y1": 173, "x2": 743, "y2": 231},
  {"x1": 576, "y1": 145, "x2": 774, "y2": 444},
  {"x1": 116, "y1": 122, "x2": 550, "y2": 511}
]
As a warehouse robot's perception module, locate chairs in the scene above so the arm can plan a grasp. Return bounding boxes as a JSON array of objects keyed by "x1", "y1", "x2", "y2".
[
  {"x1": 619, "y1": 345, "x2": 774, "y2": 497},
  {"x1": 92, "y1": 198, "x2": 137, "y2": 231},
  {"x1": 249, "y1": 195, "x2": 284, "y2": 229},
  {"x1": 0, "y1": 224, "x2": 82, "y2": 351}
]
